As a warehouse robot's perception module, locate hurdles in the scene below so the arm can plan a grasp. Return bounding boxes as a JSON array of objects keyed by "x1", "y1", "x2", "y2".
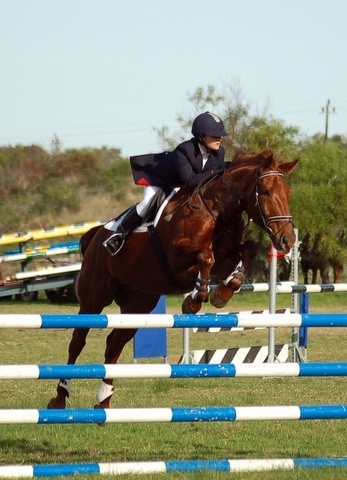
[{"x1": 0, "y1": 228, "x2": 346, "y2": 480}]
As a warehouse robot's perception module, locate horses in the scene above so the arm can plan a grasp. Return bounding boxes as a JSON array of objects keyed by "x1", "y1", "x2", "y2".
[
  {"x1": 47, "y1": 147, "x2": 299, "y2": 428},
  {"x1": 300, "y1": 234, "x2": 344, "y2": 285}
]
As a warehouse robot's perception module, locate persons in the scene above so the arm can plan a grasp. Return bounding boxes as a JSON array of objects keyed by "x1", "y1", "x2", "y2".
[{"x1": 102, "y1": 111, "x2": 225, "y2": 254}]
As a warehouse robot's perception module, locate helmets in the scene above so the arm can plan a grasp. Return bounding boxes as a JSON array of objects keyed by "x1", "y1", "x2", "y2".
[{"x1": 191, "y1": 111, "x2": 228, "y2": 136}]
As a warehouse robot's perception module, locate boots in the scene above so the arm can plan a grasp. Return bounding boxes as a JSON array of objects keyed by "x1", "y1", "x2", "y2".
[{"x1": 107, "y1": 202, "x2": 144, "y2": 253}]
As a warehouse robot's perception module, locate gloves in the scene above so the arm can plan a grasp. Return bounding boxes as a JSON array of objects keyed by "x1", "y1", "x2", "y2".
[{"x1": 215, "y1": 161, "x2": 232, "y2": 171}]
